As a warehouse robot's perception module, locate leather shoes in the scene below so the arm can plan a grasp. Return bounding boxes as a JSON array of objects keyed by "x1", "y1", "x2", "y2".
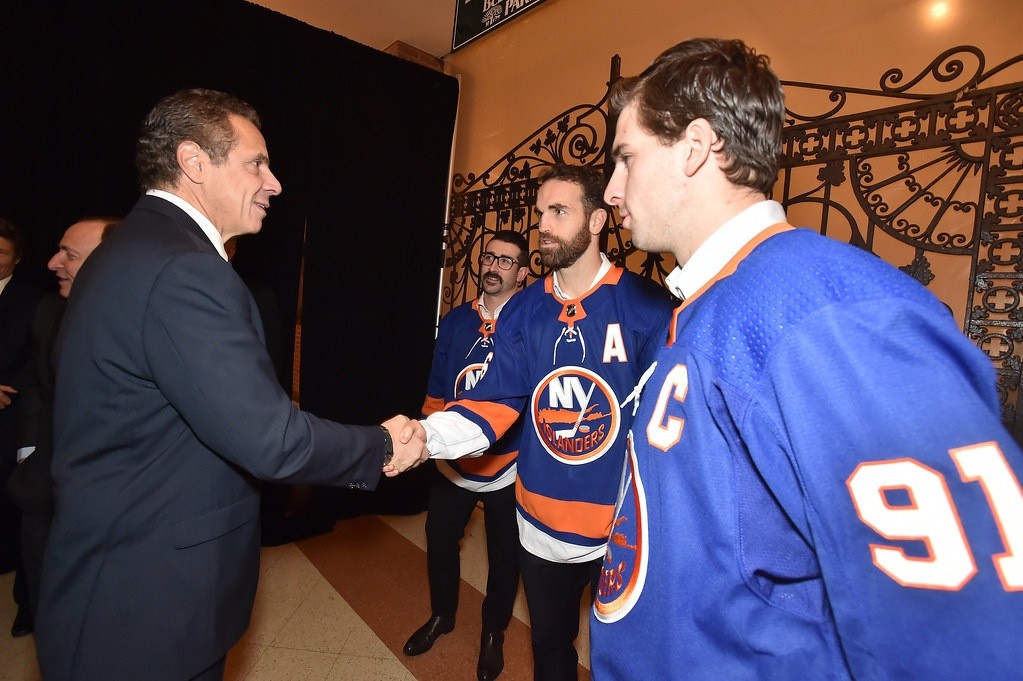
[
  {"x1": 403, "y1": 613, "x2": 455, "y2": 656},
  {"x1": 12, "y1": 606, "x2": 34, "y2": 637},
  {"x1": 476, "y1": 631, "x2": 505, "y2": 681}
]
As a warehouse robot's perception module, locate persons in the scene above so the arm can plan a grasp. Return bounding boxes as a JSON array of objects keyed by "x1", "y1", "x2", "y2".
[
  {"x1": 31, "y1": 86, "x2": 430, "y2": 681},
  {"x1": 590, "y1": 38, "x2": 1023, "y2": 681},
  {"x1": 405, "y1": 229, "x2": 523, "y2": 680},
  {"x1": 384, "y1": 166, "x2": 685, "y2": 680},
  {"x1": 0, "y1": 218, "x2": 124, "y2": 638}
]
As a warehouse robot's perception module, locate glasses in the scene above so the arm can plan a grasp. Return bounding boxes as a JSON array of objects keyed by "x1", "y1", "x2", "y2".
[{"x1": 478, "y1": 252, "x2": 521, "y2": 270}]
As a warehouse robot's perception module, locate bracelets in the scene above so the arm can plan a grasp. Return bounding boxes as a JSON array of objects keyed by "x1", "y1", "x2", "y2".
[{"x1": 378, "y1": 426, "x2": 393, "y2": 468}]
